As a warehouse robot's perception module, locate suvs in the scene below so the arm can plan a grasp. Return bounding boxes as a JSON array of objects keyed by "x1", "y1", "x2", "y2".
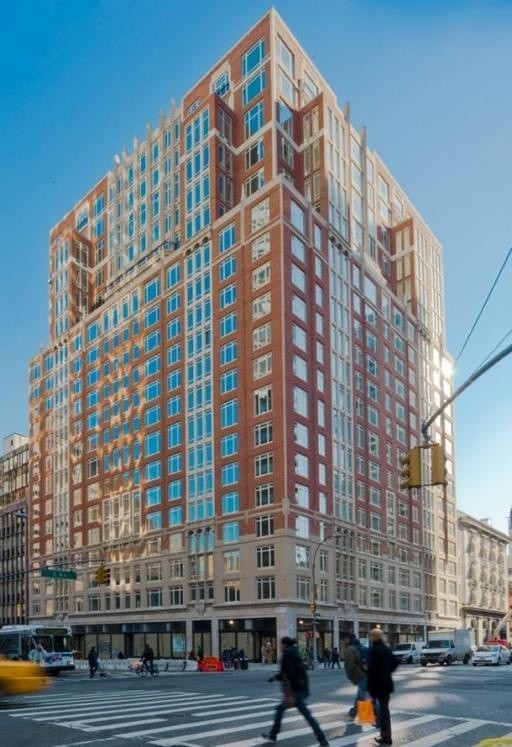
[{"x1": 392, "y1": 642, "x2": 421, "y2": 664}]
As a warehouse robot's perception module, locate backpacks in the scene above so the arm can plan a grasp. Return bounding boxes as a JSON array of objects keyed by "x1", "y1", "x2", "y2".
[{"x1": 355, "y1": 643, "x2": 370, "y2": 673}]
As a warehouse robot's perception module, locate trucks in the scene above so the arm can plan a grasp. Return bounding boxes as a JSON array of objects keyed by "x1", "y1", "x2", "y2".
[{"x1": 421, "y1": 628, "x2": 472, "y2": 667}]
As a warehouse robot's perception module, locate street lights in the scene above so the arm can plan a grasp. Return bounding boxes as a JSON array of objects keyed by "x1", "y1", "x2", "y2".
[{"x1": 311, "y1": 532, "x2": 342, "y2": 668}]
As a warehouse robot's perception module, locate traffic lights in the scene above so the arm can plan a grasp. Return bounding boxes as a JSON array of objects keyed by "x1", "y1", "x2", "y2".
[
  {"x1": 95, "y1": 568, "x2": 110, "y2": 584},
  {"x1": 400, "y1": 454, "x2": 409, "y2": 492}
]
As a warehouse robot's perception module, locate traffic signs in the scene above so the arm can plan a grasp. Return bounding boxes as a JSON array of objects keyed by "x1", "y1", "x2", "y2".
[{"x1": 40, "y1": 568, "x2": 77, "y2": 579}]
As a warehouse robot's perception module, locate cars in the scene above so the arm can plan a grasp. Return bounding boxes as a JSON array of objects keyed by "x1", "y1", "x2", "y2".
[{"x1": 473, "y1": 645, "x2": 510, "y2": 666}]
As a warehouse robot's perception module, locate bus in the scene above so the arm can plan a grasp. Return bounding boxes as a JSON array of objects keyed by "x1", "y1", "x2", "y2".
[{"x1": 0, "y1": 624, "x2": 75, "y2": 676}]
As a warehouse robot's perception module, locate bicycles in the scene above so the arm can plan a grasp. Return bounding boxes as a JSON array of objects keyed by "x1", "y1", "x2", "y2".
[{"x1": 135, "y1": 658, "x2": 159, "y2": 678}]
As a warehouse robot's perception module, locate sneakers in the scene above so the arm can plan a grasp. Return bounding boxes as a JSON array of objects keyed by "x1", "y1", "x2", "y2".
[
  {"x1": 374, "y1": 737, "x2": 392, "y2": 745},
  {"x1": 262, "y1": 732, "x2": 278, "y2": 743}
]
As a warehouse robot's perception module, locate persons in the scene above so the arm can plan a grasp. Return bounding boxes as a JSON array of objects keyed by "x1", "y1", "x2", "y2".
[
  {"x1": 362, "y1": 628, "x2": 399, "y2": 745},
  {"x1": 341, "y1": 633, "x2": 381, "y2": 729},
  {"x1": 189, "y1": 650, "x2": 196, "y2": 660},
  {"x1": 261, "y1": 642, "x2": 273, "y2": 664},
  {"x1": 88, "y1": 646, "x2": 99, "y2": 679},
  {"x1": 330, "y1": 647, "x2": 341, "y2": 669},
  {"x1": 197, "y1": 645, "x2": 204, "y2": 661},
  {"x1": 222, "y1": 646, "x2": 245, "y2": 668},
  {"x1": 118, "y1": 651, "x2": 124, "y2": 659},
  {"x1": 302, "y1": 647, "x2": 316, "y2": 670},
  {"x1": 323, "y1": 646, "x2": 331, "y2": 668},
  {"x1": 261, "y1": 636, "x2": 330, "y2": 746},
  {"x1": 140, "y1": 644, "x2": 154, "y2": 675}
]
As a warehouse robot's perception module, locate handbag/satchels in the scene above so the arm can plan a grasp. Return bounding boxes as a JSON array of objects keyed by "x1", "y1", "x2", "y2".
[{"x1": 353, "y1": 699, "x2": 377, "y2": 725}]
